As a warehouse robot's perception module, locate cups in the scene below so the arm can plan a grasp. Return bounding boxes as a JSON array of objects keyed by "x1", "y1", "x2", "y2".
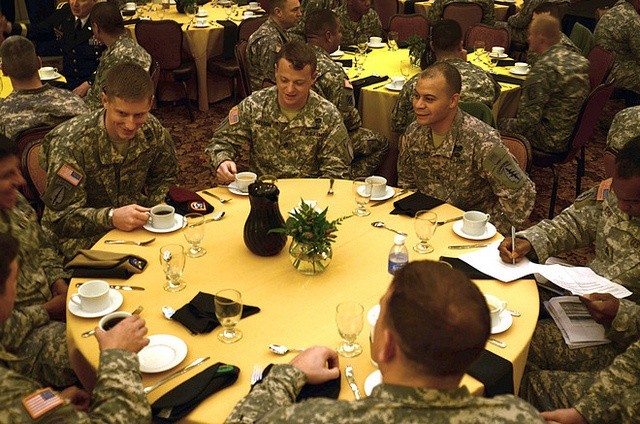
[
  {"x1": 244, "y1": 11, "x2": 255, "y2": 18},
  {"x1": 367, "y1": 303, "x2": 389, "y2": 368},
  {"x1": 196, "y1": 18, "x2": 208, "y2": 26},
  {"x1": 354, "y1": 176, "x2": 371, "y2": 217},
  {"x1": 370, "y1": 37, "x2": 382, "y2": 44},
  {"x1": 145, "y1": 204, "x2": 176, "y2": 228},
  {"x1": 391, "y1": 76, "x2": 406, "y2": 87},
  {"x1": 361, "y1": 175, "x2": 389, "y2": 198},
  {"x1": 514, "y1": 62, "x2": 530, "y2": 71},
  {"x1": 249, "y1": 2, "x2": 260, "y2": 8},
  {"x1": 482, "y1": 293, "x2": 507, "y2": 326},
  {"x1": 461, "y1": 210, "x2": 491, "y2": 235},
  {"x1": 492, "y1": 47, "x2": 505, "y2": 54},
  {"x1": 69, "y1": 280, "x2": 114, "y2": 310},
  {"x1": 39, "y1": 66, "x2": 58, "y2": 76},
  {"x1": 400, "y1": 59, "x2": 412, "y2": 79},
  {"x1": 235, "y1": 171, "x2": 257, "y2": 193},
  {"x1": 412, "y1": 209, "x2": 438, "y2": 255}
]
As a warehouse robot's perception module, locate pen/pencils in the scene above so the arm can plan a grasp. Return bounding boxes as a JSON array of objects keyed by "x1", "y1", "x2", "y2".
[{"x1": 511, "y1": 226, "x2": 516, "y2": 265}]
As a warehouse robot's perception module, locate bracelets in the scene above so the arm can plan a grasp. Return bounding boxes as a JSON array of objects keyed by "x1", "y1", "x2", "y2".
[{"x1": 107, "y1": 207, "x2": 114, "y2": 229}]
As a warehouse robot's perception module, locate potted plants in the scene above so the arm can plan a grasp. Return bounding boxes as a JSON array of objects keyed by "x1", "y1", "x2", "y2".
[{"x1": 263, "y1": 196, "x2": 354, "y2": 276}]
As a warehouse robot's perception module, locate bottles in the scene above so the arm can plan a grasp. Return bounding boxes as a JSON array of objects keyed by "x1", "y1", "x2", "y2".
[{"x1": 387, "y1": 234, "x2": 410, "y2": 291}]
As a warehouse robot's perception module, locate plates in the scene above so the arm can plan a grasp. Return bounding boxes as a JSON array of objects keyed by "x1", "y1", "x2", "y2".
[
  {"x1": 135, "y1": 334, "x2": 188, "y2": 374},
  {"x1": 39, "y1": 74, "x2": 61, "y2": 81},
  {"x1": 451, "y1": 218, "x2": 498, "y2": 241},
  {"x1": 192, "y1": 23, "x2": 210, "y2": 28},
  {"x1": 227, "y1": 181, "x2": 257, "y2": 196},
  {"x1": 508, "y1": 68, "x2": 530, "y2": 75},
  {"x1": 366, "y1": 43, "x2": 386, "y2": 49},
  {"x1": 364, "y1": 370, "x2": 385, "y2": 396},
  {"x1": 142, "y1": 210, "x2": 185, "y2": 233},
  {"x1": 68, "y1": 281, "x2": 124, "y2": 318},
  {"x1": 489, "y1": 52, "x2": 509, "y2": 58},
  {"x1": 357, "y1": 181, "x2": 395, "y2": 201},
  {"x1": 384, "y1": 83, "x2": 405, "y2": 92},
  {"x1": 489, "y1": 309, "x2": 513, "y2": 335}
]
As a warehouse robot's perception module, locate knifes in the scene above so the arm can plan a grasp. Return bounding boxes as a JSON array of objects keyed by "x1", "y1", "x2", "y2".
[
  {"x1": 370, "y1": 189, "x2": 409, "y2": 208},
  {"x1": 344, "y1": 365, "x2": 362, "y2": 401},
  {"x1": 143, "y1": 355, "x2": 210, "y2": 394},
  {"x1": 75, "y1": 283, "x2": 146, "y2": 292},
  {"x1": 448, "y1": 243, "x2": 492, "y2": 249}
]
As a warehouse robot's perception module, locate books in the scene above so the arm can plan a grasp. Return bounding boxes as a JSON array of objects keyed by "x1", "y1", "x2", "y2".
[{"x1": 542, "y1": 294, "x2": 612, "y2": 349}]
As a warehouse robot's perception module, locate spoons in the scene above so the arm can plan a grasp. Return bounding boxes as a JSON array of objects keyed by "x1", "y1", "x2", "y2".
[
  {"x1": 268, "y1": 344, "x2": 313, "y2": 355},
  {"x1": 370, "y1": 221, "x2": 407, "y2": 238},
  {"x1": 163, "y1": 304, "x2": 193, "y2": 336},
  {"x1": 189, "y1": 211, "x2": 226, "y2": 229}
]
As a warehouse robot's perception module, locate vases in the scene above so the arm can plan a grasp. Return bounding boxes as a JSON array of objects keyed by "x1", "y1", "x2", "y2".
[{"x1": 243, "y1": 181, "x2": 288, "y2": 257}]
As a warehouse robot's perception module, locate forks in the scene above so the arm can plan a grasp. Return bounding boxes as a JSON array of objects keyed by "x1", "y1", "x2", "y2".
[
  {"x1": 248, "y1": 367, "x2": 264, "y2": 386},
  {"x1": 328, "y1": 178, "x2": 335, "y2": 197},
  {"x1": 104, "y1": 237, "x2": 156, "y2": 245},
  {"x1": 202, "y1": 189, "x2": 232, "y2": 204},
  {"x1": 434, "y1": 214, "x2": 463, "y2": 226}
]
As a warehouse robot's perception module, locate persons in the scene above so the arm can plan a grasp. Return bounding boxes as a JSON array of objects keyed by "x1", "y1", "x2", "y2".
[
  {"x1": 532, "y1": 3, "x2": 583, "y2": 54},
  {"x1": 499, "y1": 15, "x2": 589, "y2": 152},
  {"x1": 427, "y1": 0, "x2": 496, "y2": 31},
  {"x1": 304, "y1": 9, "x2": 391, "y2": 182},
  {"x1": 87, "y1": 3, "x2": 151, "y2": 107},
  {"x1": 392, "y1": 18, "x2": 501, "y2": 135},
  {"x1": 42, "y1": 64, "x2": 178, "y2": 255},
  {"x1": 592, "y1": 0, "x2": 640, "y2": 95},
  {"x1": 245, "y1": 0, "x2": 301, "y2": 92},
  {"x1": 542, "y1": 333, "x2": 640, "y2": 424},
  {"x1": 327, "y1": 1, "x2": 384, "y2": 44},
  {"x1": 499, "y1": 133, "x2": 640, "y2": 370},
  {"x1": 0, "y1": 235, "x2": 152, "y2": 424},
  {"x1": 0, "y1": 0, "x2": 102, "y2": 95},
  {"x1": 0, "y1": 144, "x2": 86, "y2": 386},
  {"x1": 494, "y1": 0, "x2": 571, "y2": 45},
  {"x1": 0, "y1": 36, "x2": 86, "y2": 139},
  {"x1": 224, "y1": 258, "x2": 546, "y2": 424},
  {"x1": 396, "y1": 64, "x2": 536, "y2": 235},
  {"x1": 206, "y1": 40, "x2": 353, "y2": 183}
]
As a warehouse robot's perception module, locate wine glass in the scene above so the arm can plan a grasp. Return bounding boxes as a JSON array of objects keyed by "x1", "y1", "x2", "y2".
[
  {"x1": 354, "y1": 48, "x2": 367, "y2": 71},
  {"x1": 335, "y1": 301, "x2": 366, "y2": 357},
  {"x1": 156, "y1": 6, "x2": 166, "y2": 20},
  {"x1": 387, "y1": 30, "x2": 399, "y2": 53},
  {"x1": 474, "y1": 41, "x2": 485, "y2": 62},
  {"x1": 186, "y1": 7, "x2": 197, "y2": 28},
  {"x1": 158, "y1": 243, "x2": 187, "y2": 291},
  {"x1": 182, "y1": 213, "x2": 207, "y2": 258},
  {"x1": 214, "y1": 288, "x2": 242, "y2": 343},
  {"x1": 488, "y1": 52, "x2": 499, "y2": 75}
]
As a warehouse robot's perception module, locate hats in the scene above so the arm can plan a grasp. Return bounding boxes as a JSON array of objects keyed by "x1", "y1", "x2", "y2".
[
  {"x1": 165, "y1": 186, "x2": 214, "y2": 217},
  {"x1": 151, "y1": 362, "x2": 239, "y2": 424},
  {"x1": 64, "y1": 249, "x2": 147, "y2": 272}
]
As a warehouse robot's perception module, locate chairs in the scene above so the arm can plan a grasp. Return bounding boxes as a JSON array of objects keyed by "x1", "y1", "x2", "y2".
[
  {"x1": 16, "y1": 124, "x2": 61, "y2": 154},
  {"x1": 374, "y1": 1, "x2": 403, "y2": 31},
  {"x1": 206, "y1": 16, "x2": 270, "y2": 103},
  {"x1": 22, "y1": 140, "x2": 49, "y2": 199},
  {"x1": 533, "y1": 3, "x2": 561, "y2": 18},
  {"x1": 233, "y1": 39, "x2": 252, "y2": 98},
  {"x1": 585, "y1": 46, "x2": 617, "y2": 91},
  {"x1": 498, "y1": 130, "x2": 532, "y2": 172},
  {"x1": 134, "y1": 20, "x2": 196, "y2": 123},
  {"x1": 531, "y1": 76, "x2": 616, "y2": 220},
  {"x1": 388, "y1": 13, "x2": 429, "y2": 41},
  {"x1": 443, "y1": 2, "x2": 484, "y2": 31},
  {"x1": 606, "y1": 148, "x2": 618, "y2": 182},
  {"x1": 463, "y1": 24, "x2": 511, "y2": 51},
  {"x1": 553, "y1": 1, "x2": 570, "y2": 18}
]
